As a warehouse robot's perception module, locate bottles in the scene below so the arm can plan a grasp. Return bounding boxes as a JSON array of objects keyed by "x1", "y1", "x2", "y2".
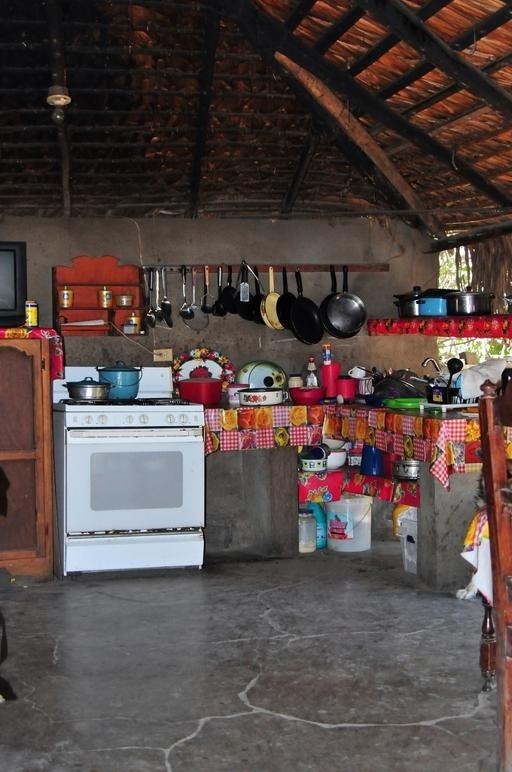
[
  {"x1": 126, "y1": 312, "x2": 141, "y2": 335},
  {"x1": 98, "y1": 286, "x2": 112, "y2": 308},
  {"x1": 227, "y1": 383, "x2": 250, "y2": 405},
  {"x1": 298, "y1": 508, "x2": 318, "y2": 554},
  {"x1": 58, "y1": 285, "x2": 73, "y2": 308},
  {"x1": 288, "y1": 344, "x2": 357, "y2": 401},
  {"x1": 22, "y1": 300, "x2": 39, "y2": 329}
]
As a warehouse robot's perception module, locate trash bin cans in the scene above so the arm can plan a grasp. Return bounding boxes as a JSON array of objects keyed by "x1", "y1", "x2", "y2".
[{"x1": 394, "y1": 507, "x2": 419, "y2": 573}]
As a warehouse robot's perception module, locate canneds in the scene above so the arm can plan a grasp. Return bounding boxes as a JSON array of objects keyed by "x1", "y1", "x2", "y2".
[{"x1": 25, "y1": 301, "x2": 40, "y2": 327}]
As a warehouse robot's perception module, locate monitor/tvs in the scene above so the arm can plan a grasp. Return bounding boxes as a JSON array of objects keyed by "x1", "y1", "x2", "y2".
[{"x1": 0, "y1": 240, "x2": 27, "y2": 328}]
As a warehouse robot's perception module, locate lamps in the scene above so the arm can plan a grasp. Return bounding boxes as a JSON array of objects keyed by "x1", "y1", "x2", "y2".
[{"x1": 46, "y1": 84, "x2": 72, "y2": 124}]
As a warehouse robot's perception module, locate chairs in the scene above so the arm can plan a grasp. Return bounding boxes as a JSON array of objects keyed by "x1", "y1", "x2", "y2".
[{"x1": 479, "y1": 379, "x2": 511, "y2": 771}]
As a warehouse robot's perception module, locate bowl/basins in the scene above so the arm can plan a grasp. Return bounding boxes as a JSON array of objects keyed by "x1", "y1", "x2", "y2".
[
  {"x1": 298, "y1": 438, "x2": 347, "y2": 472},
  {"x1": 289, "y1": 387, "x2": 327, "y2": 405},
  {"x1": 115, "y1": 296, "x2": 133, "y2": 310}
]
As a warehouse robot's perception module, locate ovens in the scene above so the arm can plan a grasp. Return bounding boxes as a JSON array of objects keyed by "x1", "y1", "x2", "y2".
[{"x1": 52, "y1": 428, "x2": 207, "y2": 576}]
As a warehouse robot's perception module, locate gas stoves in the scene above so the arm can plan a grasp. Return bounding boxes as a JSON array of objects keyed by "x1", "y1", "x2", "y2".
[{"x1": 52, "y1": 397, "x2": 205, "y2": 429}]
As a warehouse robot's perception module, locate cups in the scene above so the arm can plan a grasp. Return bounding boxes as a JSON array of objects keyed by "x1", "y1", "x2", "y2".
[{"x1": 348, "y1": 366, "x2": 366, "y2": 379}]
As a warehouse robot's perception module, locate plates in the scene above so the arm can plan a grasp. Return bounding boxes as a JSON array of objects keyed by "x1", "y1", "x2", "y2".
[
  {"x1": 173, "y1": 348, "x2": 236, "y2": 401},
  {"x1": 233, "y1": 360, "x2": 286, "y2": 388}
]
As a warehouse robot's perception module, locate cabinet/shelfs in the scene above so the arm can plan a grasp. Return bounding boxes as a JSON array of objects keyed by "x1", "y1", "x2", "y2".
[
  {"x1": 51, "y1": 254, "x2": 144, "y2": 337},
  {"x1": 0, "y1": 326, "x2": 65, "y2": 583}
]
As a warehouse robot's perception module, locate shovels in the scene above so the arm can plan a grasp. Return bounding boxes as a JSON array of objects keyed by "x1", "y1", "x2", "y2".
[{"x1": 161, "y1": 266, "x2": 170, "y2": 316}]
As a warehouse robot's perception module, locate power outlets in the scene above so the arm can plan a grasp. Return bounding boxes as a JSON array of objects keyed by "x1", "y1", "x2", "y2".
[{"x1": 153, "y1": 349, "x2": 173, "y2": 361}]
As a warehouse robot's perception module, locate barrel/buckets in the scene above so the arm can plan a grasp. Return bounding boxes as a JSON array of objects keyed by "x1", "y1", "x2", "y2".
[{"x1": 326, "y1": 492, "x2": 373, "y2": 552}]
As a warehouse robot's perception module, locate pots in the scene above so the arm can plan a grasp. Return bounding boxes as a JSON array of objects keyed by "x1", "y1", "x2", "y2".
[
  {"x1": 392, "y1": 460, "x2": 422, "y2": 479},
  {"x1": 234, "y1": 387, "x2": 289, "y2": 407},
  {"x1": 95, "y1": 359, "x2": 143, "y2": 401},
  {"x1": 392, "y1": 285, "x2": 495, "y2": 319},
  {"x1": 373, "y1": 369, "x2": 428, "y2": 401},
  {"x1": 176, "y1": 378, "x2": 226, "y2": 407},
  {"x1": 222, "y1": 259, "x2": 367, "y2": 345},
  {"x1": 61, "y1": 376, "x2": 116, "y2": 400}
]
